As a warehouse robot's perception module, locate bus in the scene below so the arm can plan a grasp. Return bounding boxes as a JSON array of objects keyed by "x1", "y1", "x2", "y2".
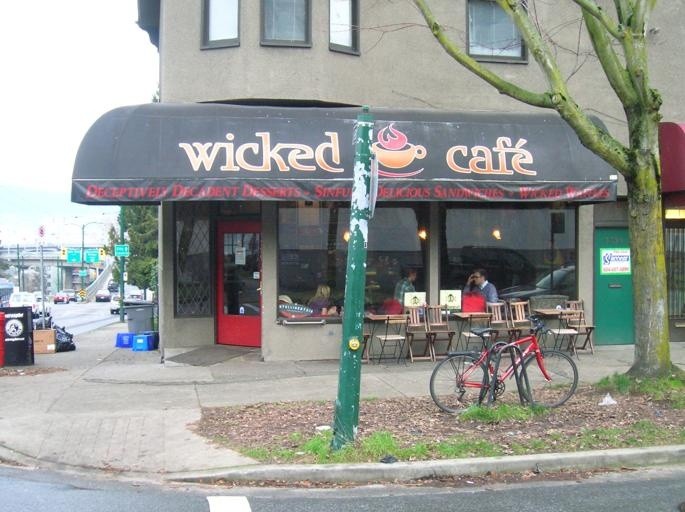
[{"x1": 0, "y1": 278, "x2": 14, "y2": 308}]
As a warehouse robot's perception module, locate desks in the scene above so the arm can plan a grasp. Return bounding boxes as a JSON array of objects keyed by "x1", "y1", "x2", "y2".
[{"x1": 366, "y1": 315, "x2": 403, "y2": 366}]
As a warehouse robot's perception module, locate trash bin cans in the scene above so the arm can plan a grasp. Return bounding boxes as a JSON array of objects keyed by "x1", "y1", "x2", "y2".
[{"x1": 123, "y1": 305, "x2": 154, "y2": 335}]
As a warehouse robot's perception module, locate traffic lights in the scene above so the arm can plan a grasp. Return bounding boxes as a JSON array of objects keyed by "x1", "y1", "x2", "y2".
[
  {"x1": 62, "y1": 248, "x2": 66, "y2": 257},
  {"x1": 100, "y1": 248, "x2": 104, "y2": 256}
]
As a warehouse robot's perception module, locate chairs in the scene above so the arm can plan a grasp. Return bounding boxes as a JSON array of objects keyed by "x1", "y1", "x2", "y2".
[{"x1": 377, "y1": 298, "x2": 595, "y2": 363}]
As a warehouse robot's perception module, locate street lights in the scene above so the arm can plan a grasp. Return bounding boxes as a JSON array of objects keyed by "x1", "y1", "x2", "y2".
[{"x1": 66, "y1": 221, "x2": 108, "y2": 301}]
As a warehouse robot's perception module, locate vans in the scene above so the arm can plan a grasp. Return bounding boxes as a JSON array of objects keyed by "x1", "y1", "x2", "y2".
[
  {"x1": 110, "y1": 296, "x2": 127, "y2": 314},
  {"x1": 9, "y1": 291, "x2": 40, "y2": 317}
]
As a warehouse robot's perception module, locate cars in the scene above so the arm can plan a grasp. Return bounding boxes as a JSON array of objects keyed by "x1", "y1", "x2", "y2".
[
  {"x1": 129, "y1": 294, "x2": 143, "y2": 301},
  {"x1": 54, "y1": 292, "x2": 70, "y2": 304},
  {"x1": 444, "y1": 263, "x2": 476, "y2": 290},
  {"x1": 108, "y1": 278, "x2": 120, "y2": 291},
  {"x1": 500, "y1": 262, "x2": 578, "y2": 311},
  {"x1": 96, "y1": 289, "x2": 111, "y2": 302},
  {"x1": 35, "y1": 294, "x2": 51, "y2": 316}
]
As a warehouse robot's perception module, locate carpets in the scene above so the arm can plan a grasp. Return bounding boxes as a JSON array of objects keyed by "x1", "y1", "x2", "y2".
[{"x1": 167, "y1": 345, "x2": 257, "y2": 367}]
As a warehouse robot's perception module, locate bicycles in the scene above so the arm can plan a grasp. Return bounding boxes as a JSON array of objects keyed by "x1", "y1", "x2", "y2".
[{"x1": 428, "y1": 314, "x2": 579, "y2": 417}]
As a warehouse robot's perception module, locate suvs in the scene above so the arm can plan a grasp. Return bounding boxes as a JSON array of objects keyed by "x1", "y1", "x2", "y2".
[{"x1": 63, "y1": 289, "x2": 77, "y2": 302}]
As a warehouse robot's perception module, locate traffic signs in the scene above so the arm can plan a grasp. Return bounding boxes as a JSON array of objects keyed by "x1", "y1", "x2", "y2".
[
  {"x1": 67, "y1": 250, "x2": 79, "y2": 262},
  {"x1": 84, "y1": 249, "x2": 98, "y2": 261}
]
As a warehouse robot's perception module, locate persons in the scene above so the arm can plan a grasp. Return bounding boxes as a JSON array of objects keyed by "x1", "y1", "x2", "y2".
[
  {"x1": 394, "y1": 266, "x2": 419, "y2": 305},
  {"x1": 306, "y1": 283, "x2": 334, "y2": 314},
  {"x1": 463, "y1": 267, "x2": 500, "y2": 303}
]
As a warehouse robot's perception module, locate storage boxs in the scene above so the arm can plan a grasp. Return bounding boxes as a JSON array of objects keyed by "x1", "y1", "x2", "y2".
[
  {"x1": 115, "y1": 332, "x2": 136, "y2": 348},
  {"x1": 132, "y1": 334, "x2": 153, "y2": 350},
  {"x1": 33, "y1": 328, "x2": 57, "y2": 355},
  {"x1": 140, "y1": 331, "x2": 159, "y2": 349}
]
